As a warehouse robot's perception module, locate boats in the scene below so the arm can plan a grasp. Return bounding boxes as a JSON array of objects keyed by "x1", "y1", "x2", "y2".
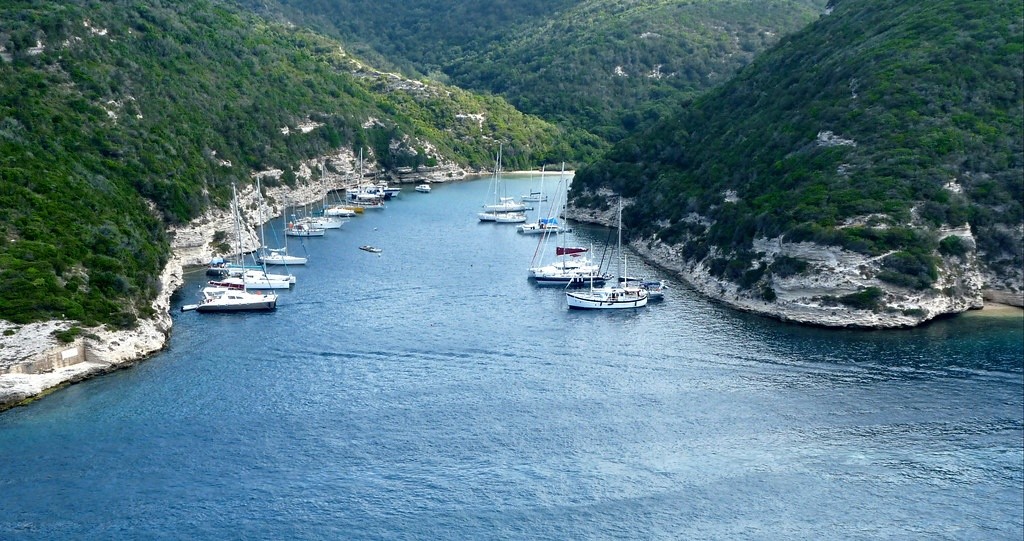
[
  {"x1": 521, "y1": 166, "x2": 548, "y2": 202},
  {"x1": 415, "y1": 184, "x2": 432, "y2": 193},
  {"x1": 359, "y1": 245, "x2": 382, "y2": 252},
  {"x1": 194, "y1": 146, "x2": 403, "y2": 313}
]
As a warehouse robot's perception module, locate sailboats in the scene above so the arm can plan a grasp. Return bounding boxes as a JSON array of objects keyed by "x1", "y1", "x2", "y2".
[
  {"x1": 516, "y1": 164, "x2": 559, "y2": 233},
  {"x1": 602, "y1": 193, "x2": 666, "y2": 301},
  {"x1": 475, "y1": 143, "x2": 534, "y2": 223},
  {"x1": 565, "y1": 237, "x2": 648, "y2": 310},
  {"x1": 526, "y1": 161, "x2": 614, "y2": 286}
]
